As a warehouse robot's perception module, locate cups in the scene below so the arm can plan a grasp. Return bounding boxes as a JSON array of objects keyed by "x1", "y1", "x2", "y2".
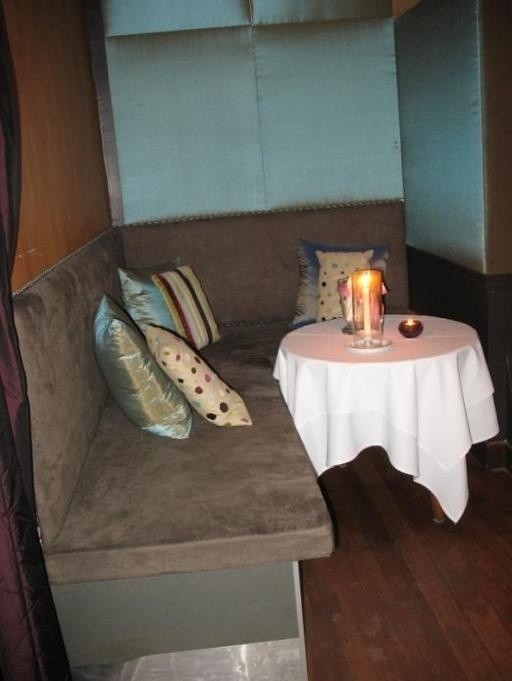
[{"x1": 399, "y1": 320, "x2": 423, "y2": 338}]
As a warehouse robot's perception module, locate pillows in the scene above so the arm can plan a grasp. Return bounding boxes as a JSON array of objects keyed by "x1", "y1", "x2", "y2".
[
  {"x1": 314, "y1": 249, "x2": 378, "y2": 322},
  {"x1": 288, "y1": 237, "x2": 389, "y2": 329},
  {"x1": 93, "y1": 256, "x2": 252, "y2": 441}
]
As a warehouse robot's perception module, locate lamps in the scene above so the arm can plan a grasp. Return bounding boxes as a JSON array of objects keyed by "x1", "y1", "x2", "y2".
[{"x1": 348, "y1": 269, "x2": 393, "y2": 349}]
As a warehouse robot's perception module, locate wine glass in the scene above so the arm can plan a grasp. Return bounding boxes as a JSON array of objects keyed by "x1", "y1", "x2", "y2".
[{"x1": 338, "y1": 277, "x2": 354, "y2": 335}]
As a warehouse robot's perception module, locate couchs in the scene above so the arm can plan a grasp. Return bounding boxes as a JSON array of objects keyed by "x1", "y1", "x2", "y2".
[{"x1": 11, "y1": 199, "x2": 411, "y2": 668}]
